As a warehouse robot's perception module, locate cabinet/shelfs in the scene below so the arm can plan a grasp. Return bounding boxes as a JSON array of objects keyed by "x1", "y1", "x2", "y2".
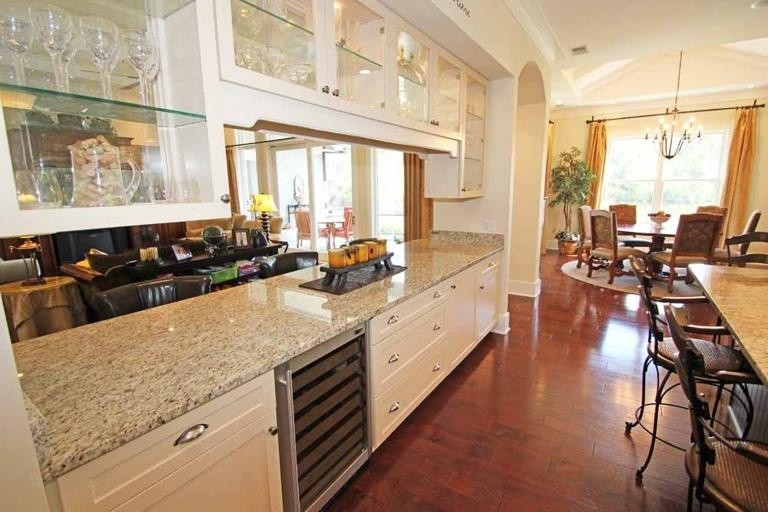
[
  {"x1": 447, "y1": 267, "x2": 475, "y2": 378},
  {"x1": 369, "y1": 282, "x2": 446, "y2": 449},
  {"x1": 217, "y1": 2, "x2": 388, "y2": 120},
  {"x1": 474, "y1": 246, "x2": 503, "y2": 347},
  {"x1": 2, "y1": 1, "x2": 234, "y2": 233},
  {"x1": 424, "y1": 69, "x2": 491, "y2": 198},
  {"x1": 46, "y1": 374, "x2": 286, "y2": 512},
  {"x1": 389, "y1": 17, "x2": 461, "y2": 134}
]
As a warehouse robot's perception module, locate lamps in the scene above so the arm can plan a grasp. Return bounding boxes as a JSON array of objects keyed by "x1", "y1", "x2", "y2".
[
  {"x1": 247, "y1": 192, "x2": 279, "y2": 242},
  {"x1": 644, "y1": 50, "x2": 703, "y2": 162}
]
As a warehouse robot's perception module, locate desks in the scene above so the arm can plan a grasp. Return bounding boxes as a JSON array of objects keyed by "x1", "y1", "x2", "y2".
[
  {"x1": 0, "y1": 275, "x2": 92, "y2": 342},
  {"x1": 688, "y1": 262, "x2": 768, "y2": 393},
  {"x1": 272, "y1": 240, "x2": 290, "y2": 253},
  {"x1": 119, "y1": 239, "x2": 288, "y2": 296}
]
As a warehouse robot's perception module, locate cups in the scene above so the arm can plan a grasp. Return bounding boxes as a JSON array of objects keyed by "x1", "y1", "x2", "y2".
[
  {"x1": 329, "y1": 239, "x2": 388, "y2": 268},
  {"x1": 64, "y1": 144, "x2": 140, "y2": 207}
]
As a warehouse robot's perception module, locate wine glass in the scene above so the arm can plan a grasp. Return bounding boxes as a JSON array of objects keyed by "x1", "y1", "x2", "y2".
[
  {"x1": 78, "y1": 15, "x2": 118, "y2": 98},
  {"x1": 57, "y1": 16, "x2": 81, "y2": 93},
  {"x1": 29, "y1": 6, "x2": 70, "y2": 92},
  {"x1": 1, "y1": 16, "x2": 36, "y2": 87},
  {"x1": 121, "y1": 26, "x2": 158, "y2": 110}
]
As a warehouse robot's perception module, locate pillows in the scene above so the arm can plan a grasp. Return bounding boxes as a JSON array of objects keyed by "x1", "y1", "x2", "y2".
[
  {"x1": 75, "y1": 246, "x2": 109, "y2": 272},
  {"x1": 232, "y1": 214, "x2": 247, "y2": 229},
  {"x1": 86, "y1": 248, "x2": 139, "y2": 274},
  {"x1": 184, "y1": 228, "x2": 204, "y2": 237}
]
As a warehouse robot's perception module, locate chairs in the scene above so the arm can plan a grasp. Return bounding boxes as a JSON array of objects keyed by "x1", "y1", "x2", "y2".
[
  {"x1": 623, "y1": 253, "x2": 754, "y2": 481},
  {"x1": 287, "y1": 203, "x2": 353, "y2": 251},
  {"x1": 709, "y1": 230, "x2": 768, "y2": 349},
  {"x1": 260, "y1": 251, "x2": 319, "y2": 279},
  {"x1": 93, "y1": 274, "x2": 214, "y2": 321},
  {"x1": 661, "y1": 299, "x2": 767, "y2": 512},
  {"x1": 573, "y1": 202, "x2": 763, "y2": 295}
]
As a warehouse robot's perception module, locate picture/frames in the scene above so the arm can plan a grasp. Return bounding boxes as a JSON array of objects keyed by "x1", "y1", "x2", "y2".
[
  {"x1": 232, "y1": 227, "x2": 251, "y2": 251},
  {"x1": 170, "y1": 241, "x2": 194, "y2": 262}
]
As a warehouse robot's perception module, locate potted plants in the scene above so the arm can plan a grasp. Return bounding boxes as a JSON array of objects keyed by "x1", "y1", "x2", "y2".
[{"x1": 548, "y1": 146, "x2": 599, "y2": 257}]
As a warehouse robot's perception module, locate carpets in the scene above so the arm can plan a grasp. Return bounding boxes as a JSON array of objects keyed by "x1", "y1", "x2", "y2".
[{"x1": 560, "y1": 258, "x2": 705, "y2": 299}]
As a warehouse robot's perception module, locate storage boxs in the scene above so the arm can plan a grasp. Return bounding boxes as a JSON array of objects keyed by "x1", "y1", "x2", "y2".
[
  {"x1": 236, "y1": 262, "x2": 256, "y2": 278},
  {"x1": 194, "y1": 262, "x2": 239, "y2": 285}
]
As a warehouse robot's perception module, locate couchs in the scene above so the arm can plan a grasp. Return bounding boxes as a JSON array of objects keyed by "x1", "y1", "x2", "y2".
[
  {"x1": 60, "y1": 231, "x2": 227, "y2": 314},
  {"x1": 179, "y1": 213, "x2": 284, "y2": 240}
]
